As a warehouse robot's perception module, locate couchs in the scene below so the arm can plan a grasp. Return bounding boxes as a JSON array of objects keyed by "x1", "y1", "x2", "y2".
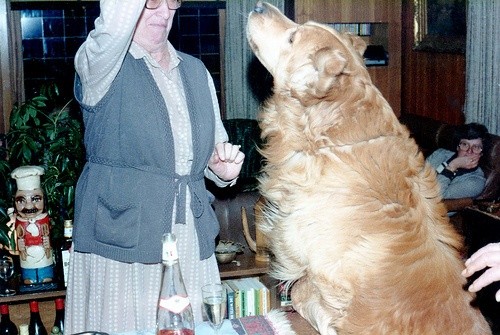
[{"x1": 400, "y1": 113, "x2": 500, "y2": 239}]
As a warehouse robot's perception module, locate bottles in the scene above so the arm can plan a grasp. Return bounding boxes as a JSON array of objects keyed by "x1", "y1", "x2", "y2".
[
  {"x1": 28, "y1": 301, "x2": 48, "y2": 335},
  {"x1": 155, "y1": 232, "x2": 195, "y2": 335},
  {"x1": 0, "y1": 304, "x2": 19, "y2": 335},
  {"x1": 55, "y1": 220, "x2": 74, "y2": 291},
  {"x1": 49, "y1": 298, "x2": 65, "y2": 335}
]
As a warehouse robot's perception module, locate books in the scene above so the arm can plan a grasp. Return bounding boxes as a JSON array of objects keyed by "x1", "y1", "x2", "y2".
[{"x1": 221, "y1": 277, "x2": 272, "y2": 320}]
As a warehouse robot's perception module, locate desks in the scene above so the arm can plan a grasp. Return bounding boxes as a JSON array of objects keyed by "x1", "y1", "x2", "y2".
[{"x1": 461, "y1": 201, "x2": 500, "y2": 335}]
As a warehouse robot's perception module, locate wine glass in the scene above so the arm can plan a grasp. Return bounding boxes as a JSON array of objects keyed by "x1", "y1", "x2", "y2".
[
  {"x1": 0, "y1": 256, "x2": 16, "y2": 297},
  {"x1": 201, "y1": 284, "x2": 228, "y2": 335}
]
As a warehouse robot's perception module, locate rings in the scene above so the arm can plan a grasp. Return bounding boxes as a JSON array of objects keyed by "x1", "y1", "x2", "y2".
[{"x1": 470, "y1": 159, "x2": 473, "y2": 162}]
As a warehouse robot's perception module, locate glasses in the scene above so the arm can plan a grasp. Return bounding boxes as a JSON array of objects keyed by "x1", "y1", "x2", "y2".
[
  {"x1": 145, "y1": 0, "x2": 182, "y2": 10},
  {"x1": 459, "y1": 141, "x2": 483, "y2": 154}
]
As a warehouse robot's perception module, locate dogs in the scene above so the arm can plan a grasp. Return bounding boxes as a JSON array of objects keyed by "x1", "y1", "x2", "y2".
[{"x1": 243, "y1": 2, "x2": 491, "y2": 335}]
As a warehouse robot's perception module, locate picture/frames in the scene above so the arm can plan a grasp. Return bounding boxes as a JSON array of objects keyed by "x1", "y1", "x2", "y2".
[{"x1": 411, "y1": 0, "x2": 467, "y2": 55}]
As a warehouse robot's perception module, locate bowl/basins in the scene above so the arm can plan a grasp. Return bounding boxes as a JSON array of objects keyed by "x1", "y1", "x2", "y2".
[{"x1": 214, "y1": 251, "x2": 237, "y2": 263}]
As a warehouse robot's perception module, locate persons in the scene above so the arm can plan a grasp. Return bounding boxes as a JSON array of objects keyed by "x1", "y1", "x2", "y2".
[
  {"x1": 11, "y1": 167, "x2": 57, "y2": 294},
  {"x1": 63, "y1": 0, "x2": 246, "y2": 335},
  {"x1": 461, "y1": 240, "x2": 500, "y2": 306},
  {"x1": 427, "y1": 122, "x2": 488, "y2": 198}
]
